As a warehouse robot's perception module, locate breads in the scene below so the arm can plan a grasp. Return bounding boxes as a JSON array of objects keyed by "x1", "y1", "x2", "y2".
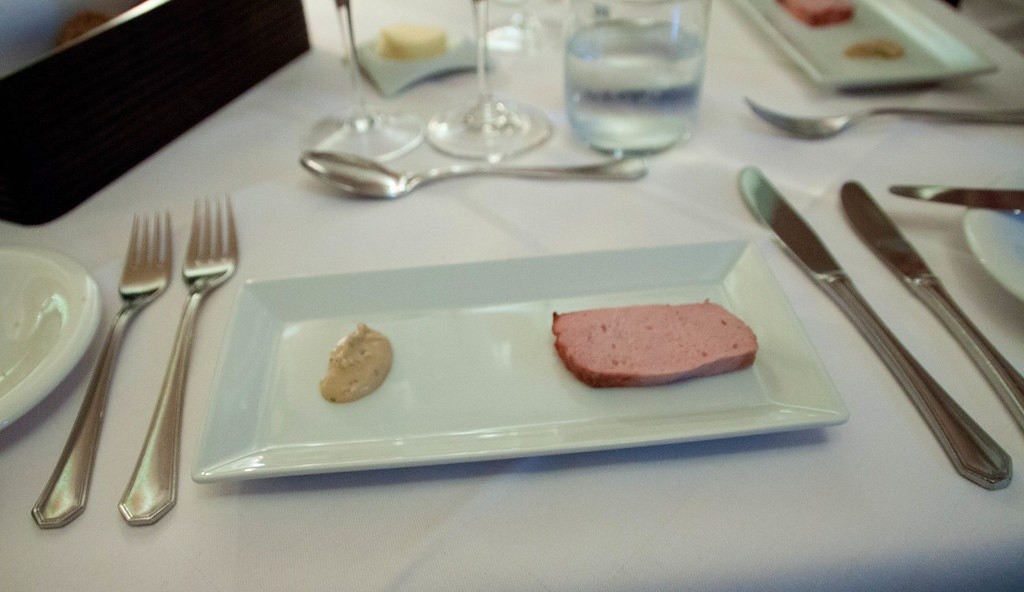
[
  {"x1": 553, "y1": 302, "x2": 758, "y2": 387},
  {"x1": 777, "y1": 0, "x2": 854, "y2": 25}
]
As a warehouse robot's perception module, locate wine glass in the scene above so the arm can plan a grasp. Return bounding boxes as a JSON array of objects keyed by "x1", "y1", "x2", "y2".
[
  {"x1": 303, "y1": 0, "x2": 424, "y2": 165},
  {"x1": 425, "y1": 0, "x2": 555, "y2": 157}
]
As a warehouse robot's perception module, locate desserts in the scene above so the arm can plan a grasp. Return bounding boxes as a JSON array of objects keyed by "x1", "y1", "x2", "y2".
[{"x1": 380, "y1": 23, "x2": 449, "y2": 60}]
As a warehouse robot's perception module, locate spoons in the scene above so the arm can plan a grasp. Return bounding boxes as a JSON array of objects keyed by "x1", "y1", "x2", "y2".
[{"x1": 297, "y1": 150, "x2": 649, "y2": 199}]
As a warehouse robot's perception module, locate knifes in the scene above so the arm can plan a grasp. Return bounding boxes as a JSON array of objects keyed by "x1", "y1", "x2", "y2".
[
  {"x1": 839, "y1": 180, "x2": 1024, "y2": 433},
  {"x1": 888, "y1": 185, "x2": 1023, "y2": 211},
  {"x1": 738, "y1": 167, "x2": 1012, "y2": 491}
]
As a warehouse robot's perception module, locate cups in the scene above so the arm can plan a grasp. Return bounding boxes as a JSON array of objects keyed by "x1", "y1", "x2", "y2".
[{"x1": 565, "y1": 0, "x2": 710, "y2": 155}]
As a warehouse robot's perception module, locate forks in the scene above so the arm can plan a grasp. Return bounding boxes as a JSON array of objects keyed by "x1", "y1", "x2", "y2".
[
  {"x1": 120, "y1": 192, "x2": 237, "y2": 525},
  {"x1": 30, "y1": 212, "x2": 172, "y2": 530},
  {"x1": 742, "y1": 96, "x2": 1023, "y2": 138}
]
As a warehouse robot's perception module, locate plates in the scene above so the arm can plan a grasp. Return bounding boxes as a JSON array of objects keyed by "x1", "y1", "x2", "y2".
[
  {"x1": 0, "y1": 248, "x2": 101, "y2": 431},
  {"x1": 192, "y1": 240, "x2": 846, "y2": 485},
  {"x1": 734, "y1": 0, "x2": 998, "y2": 92},
  {"x1": 961, "y1": 206, "x2": 1024, "y2": 301},
  {"x1": 358, "y1": 35, "x2": 486, "y2": 96}
]
